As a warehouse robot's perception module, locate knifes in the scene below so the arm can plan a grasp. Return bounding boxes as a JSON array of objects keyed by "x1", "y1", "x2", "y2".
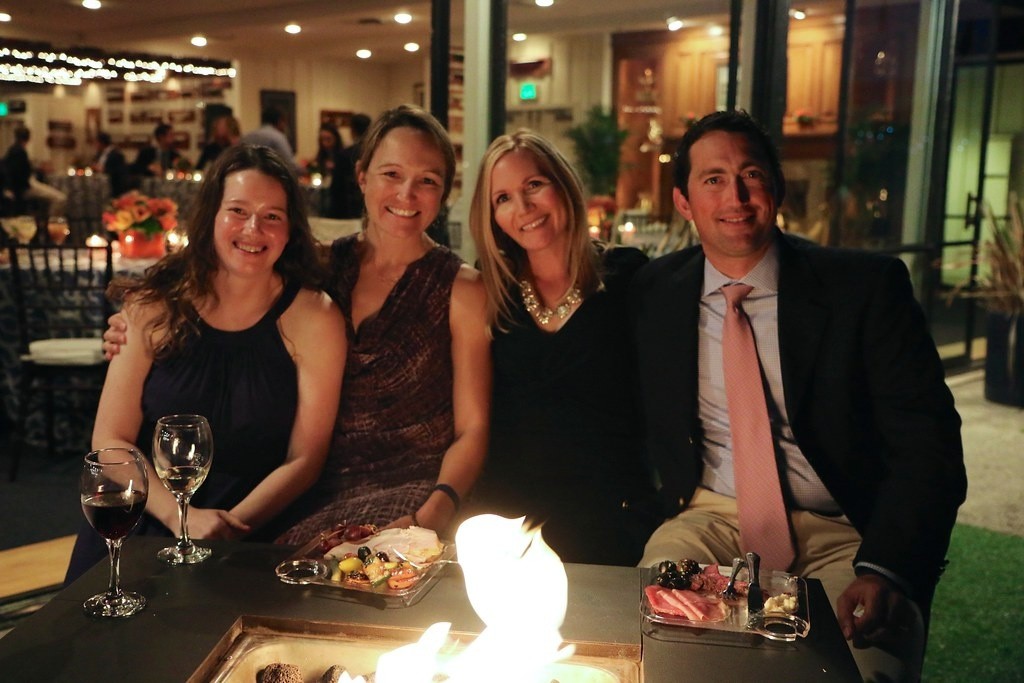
[{"x1": 746, "y1": 552, "x2": 763, "y2": 613}]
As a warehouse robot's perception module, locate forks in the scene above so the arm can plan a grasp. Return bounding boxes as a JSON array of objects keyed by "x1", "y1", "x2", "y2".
[{"x1": 718, "y1": 557, "x2": 745, "y2": 601}]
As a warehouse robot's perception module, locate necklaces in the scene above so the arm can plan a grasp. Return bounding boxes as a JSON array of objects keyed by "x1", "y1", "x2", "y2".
[{"x1": 520, "y1": 271, "x2": 581, "y2": 324}]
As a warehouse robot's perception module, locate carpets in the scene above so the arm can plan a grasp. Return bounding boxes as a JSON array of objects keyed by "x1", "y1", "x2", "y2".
[{"x1": 921, "y1": 522, "x2": 1024, "y2": 683}]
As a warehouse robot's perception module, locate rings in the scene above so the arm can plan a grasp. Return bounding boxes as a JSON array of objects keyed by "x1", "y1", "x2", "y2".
[{"x1": 900, "y1": 626, "x2": 909, "y2": 631}]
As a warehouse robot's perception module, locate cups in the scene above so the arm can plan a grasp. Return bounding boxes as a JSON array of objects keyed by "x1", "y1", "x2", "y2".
[{"x1": 47, "y1": 216, "x2": 69, "y2": 246}]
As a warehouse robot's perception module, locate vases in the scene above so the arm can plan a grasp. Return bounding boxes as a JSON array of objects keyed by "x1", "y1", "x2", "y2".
[{"x1": 118, "y1": 229, "x2": 165, "y2": 258}]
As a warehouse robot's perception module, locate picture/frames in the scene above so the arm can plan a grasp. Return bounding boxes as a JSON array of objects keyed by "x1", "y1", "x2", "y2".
[{"x1": 412, "y1": 81, "x2": 425, "y2": 109}]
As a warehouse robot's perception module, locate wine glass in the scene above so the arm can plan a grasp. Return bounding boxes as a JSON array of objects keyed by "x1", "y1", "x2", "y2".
[
  {"x1": 79, "y1": 447, "x2": 150, "y2": 623},
  {"x1": 152, "y1": 414, "x2": 214, "y2": 566}
]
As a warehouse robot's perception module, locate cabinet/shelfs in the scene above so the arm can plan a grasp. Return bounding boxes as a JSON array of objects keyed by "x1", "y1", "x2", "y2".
[{"x1": 617, "y1": 18, "x2": 844, "y2": 225}]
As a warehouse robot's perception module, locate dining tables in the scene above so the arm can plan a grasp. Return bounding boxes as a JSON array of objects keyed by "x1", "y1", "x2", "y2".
[
  {"x1": 0, "y1": 533, "x2": 865, "y2": 683},
  {"x1": 0, "y1": 248, "x2": 180, "y2": 454}
]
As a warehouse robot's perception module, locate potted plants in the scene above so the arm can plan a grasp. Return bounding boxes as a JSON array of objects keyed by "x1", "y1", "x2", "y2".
[
  {"x1": 838, "y1": 118, "x2": 899, "y2": 231},
  {"x1": 563, "y1": 103, "x2": 633, "y2": 242},
  {"x1": 984, "y1": 190, "x2": 1024, "y2": 410}
]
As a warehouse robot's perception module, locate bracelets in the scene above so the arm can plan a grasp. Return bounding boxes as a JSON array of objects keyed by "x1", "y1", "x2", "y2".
[
  {"x1": 433, "y1": 484, "x2": 460, "y2": 512},
  {"x1": 411, "y1": 511, "x2": 418, "y2": 527}
]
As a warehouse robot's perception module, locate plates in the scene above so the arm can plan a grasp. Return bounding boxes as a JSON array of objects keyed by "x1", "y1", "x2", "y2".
[
  {"x1": 275, "y1": 523, "x2": 456, "y2": 597},
  {"x1": 639, "y1": 560, "x2": 811, "y2": 642}
]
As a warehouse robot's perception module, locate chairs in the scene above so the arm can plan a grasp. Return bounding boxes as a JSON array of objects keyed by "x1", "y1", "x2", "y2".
[{"x1": 7, "y1": 236, "x2": 119, "y2": 456}]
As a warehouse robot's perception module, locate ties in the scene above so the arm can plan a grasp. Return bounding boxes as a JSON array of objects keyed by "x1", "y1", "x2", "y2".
[{"x1": 719, "y1": 283, "x2": 796, "y2": 575}]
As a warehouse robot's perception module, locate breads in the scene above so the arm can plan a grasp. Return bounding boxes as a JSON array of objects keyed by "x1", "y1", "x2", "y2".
[
  {"x1": 321, "y1": 665, "x2": 346, "y2": 683},
  {"x1": 259, "y1": 663, "x2": 303, "y2": 683}
]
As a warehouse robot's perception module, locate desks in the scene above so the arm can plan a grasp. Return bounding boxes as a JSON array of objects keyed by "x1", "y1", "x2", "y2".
[
  {"x1": 145, "y1": 177, "x2": 202, "y2": 239},
  {"x1": 307, "y1": 217, "x2": 364, "y2": 269},
  {"x1": 300, "y1": 180, "x2": 328, "y2": 218},
  {"x1": 47, "y1": 173, "x2": 108, "y2": 236}
]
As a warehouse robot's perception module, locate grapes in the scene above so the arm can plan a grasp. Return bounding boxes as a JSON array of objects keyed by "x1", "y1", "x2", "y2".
[{"x1": 320, "y1": 523, "x2": 374, "y2": 555}]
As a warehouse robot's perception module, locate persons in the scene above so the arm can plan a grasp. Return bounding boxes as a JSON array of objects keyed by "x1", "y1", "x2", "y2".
[
  {"x1": 63, "y1": 143, "x2": 348, "y2": 588},
  {"x1": 246, "y1": 108, "x2": 294, "y2": 166},
  {"x1": 631, "y1": 111, "x2": 967, "y2": 683},
  {"x1": 308, "y1": 114, "x2": 371, "y2": 218},
  {"x1": 471, "y1": 129, "x2": 648, "y2": 565},
  {"x1": 103, "y1": 101, "x2": 492, "y2": 532},
  {"x1": 0, "y1": 125, "x2": 33, "y2": 195},
  {"x1": 94, "y1": 116, "x2": 241, "y2": 197}
]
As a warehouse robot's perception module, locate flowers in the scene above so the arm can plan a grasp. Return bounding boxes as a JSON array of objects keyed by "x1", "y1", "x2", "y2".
[{"x1": 102, "y1": 191, "x2": 178, "y2": 241}]
